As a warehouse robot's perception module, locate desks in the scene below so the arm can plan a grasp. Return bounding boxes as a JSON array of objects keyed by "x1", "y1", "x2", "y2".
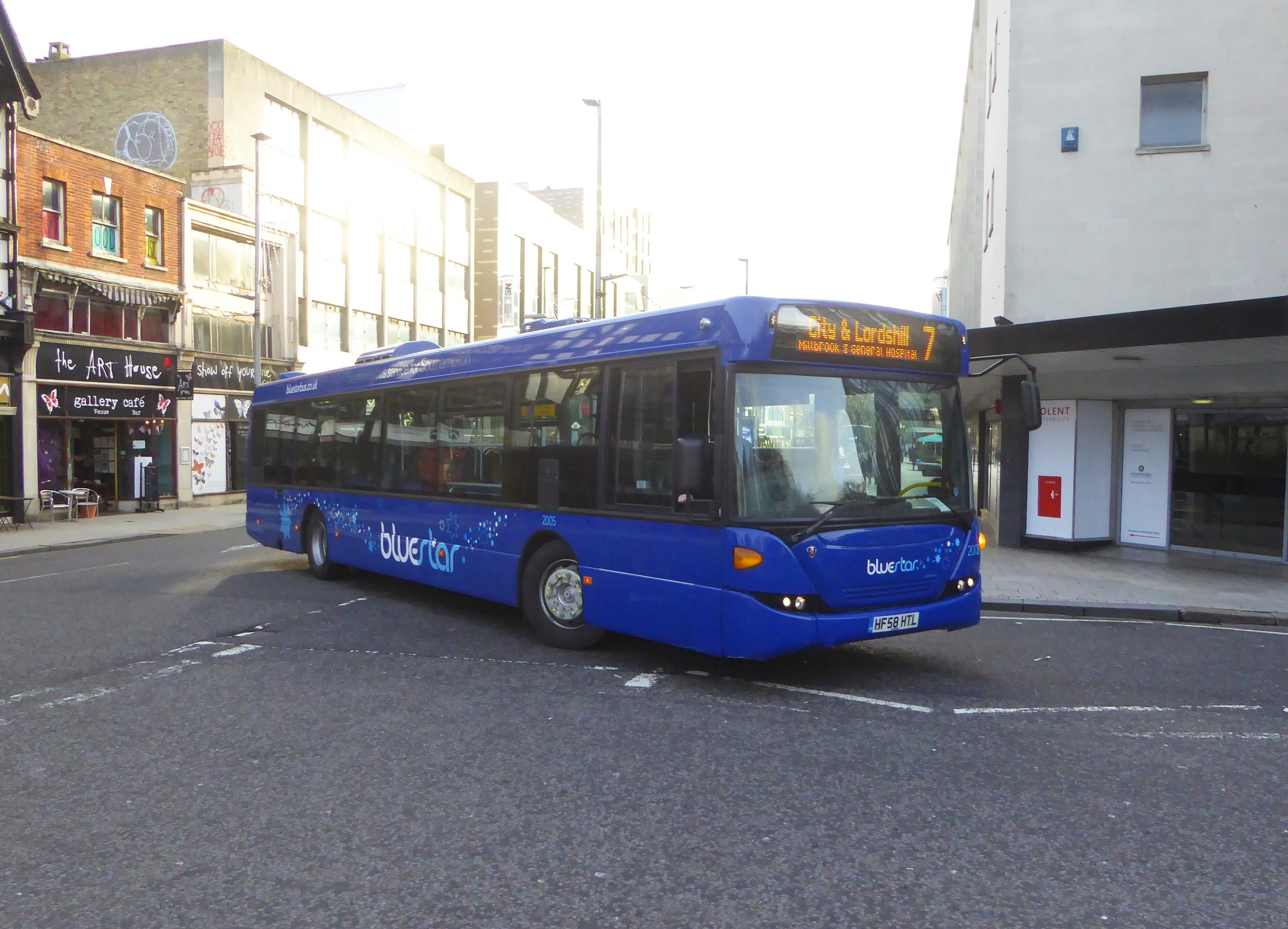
[
  {"x1": 58, "y1": 490, "x2": 85, "y2": 522},
  {"x1": 0, "y1": 496, "x2": 34, "y2": 532},
  {"x1": 82, "y1": 483, "x2": 108, "y2": 493}
]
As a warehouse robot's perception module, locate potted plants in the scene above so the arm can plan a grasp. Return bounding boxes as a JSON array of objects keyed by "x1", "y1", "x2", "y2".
[{"x1": 78, "y1": 493, "x2": 104, "y2": 518}]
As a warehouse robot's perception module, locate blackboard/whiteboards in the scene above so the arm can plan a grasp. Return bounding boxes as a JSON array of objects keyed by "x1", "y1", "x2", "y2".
[{"x1": 144, "y1": 462, "x2": 160, "y2": 502}]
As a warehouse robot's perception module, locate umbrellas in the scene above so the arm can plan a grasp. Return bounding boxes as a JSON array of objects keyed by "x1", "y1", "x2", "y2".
[{"x1": 916, "y1": 433, "x2": 943, "y2": 457}]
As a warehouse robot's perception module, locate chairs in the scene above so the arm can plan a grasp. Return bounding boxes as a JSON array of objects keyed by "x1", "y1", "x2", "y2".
[
  {"x1": 0, "y1": 500, "x2": 16, "y2": 530},
  {"x1": 37, "y1": 490, "x2": 70, "y2": 524},
  {"x1": 73, "y1": 488, "x2": 99, "y2": 519},
  {"x1": 369, "y1": 442, "x2": 633, "y2": 513}
]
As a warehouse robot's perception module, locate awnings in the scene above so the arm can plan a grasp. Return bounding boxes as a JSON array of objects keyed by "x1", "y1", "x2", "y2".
[{"x1": 33, "y1": 270, "x2": 182, "y2": 324}]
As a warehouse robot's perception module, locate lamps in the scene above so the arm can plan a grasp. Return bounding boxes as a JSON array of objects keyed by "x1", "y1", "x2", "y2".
[{"x1": 993, "y1": 316, "x2": 1013, "y2": 327}]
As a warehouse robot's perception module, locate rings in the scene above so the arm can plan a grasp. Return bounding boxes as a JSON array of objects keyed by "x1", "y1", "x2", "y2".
[{"x1": 682, "y1": 498, "x2": 683, "y2": 499}]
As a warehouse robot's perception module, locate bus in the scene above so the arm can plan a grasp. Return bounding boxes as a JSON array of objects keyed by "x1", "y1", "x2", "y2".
[{"x1": 243, "y1": 296, "x2": 1042, "y2": 659}]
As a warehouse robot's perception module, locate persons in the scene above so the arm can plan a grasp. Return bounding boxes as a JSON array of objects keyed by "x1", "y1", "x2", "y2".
[
  {"x1": 74, "y1": 447, "x2": 86, "y2": 487},
  {"x1": 907, "y1": 446, "x2": 916, "y2": 470},
  {"x1": 858, "y1": 451, "x2": 877, "y2": 485},
  {"x1": 443, "y1": 458, "x2": 456, "y2": 482},
  {"x1": 678, "y1": 437, "x2": 752, "y2": 502}
]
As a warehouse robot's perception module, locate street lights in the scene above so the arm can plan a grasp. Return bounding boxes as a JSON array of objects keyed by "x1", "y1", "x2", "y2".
[
  {"x1": 583, "y1": 99, "x2": 602, "y2": 319},
  {"x1": 250, "y1": 131, "x2": 273, "y2": 384},
  {"x1": 738, "y1": 257, "x2": 749, "y2": 295}
]
